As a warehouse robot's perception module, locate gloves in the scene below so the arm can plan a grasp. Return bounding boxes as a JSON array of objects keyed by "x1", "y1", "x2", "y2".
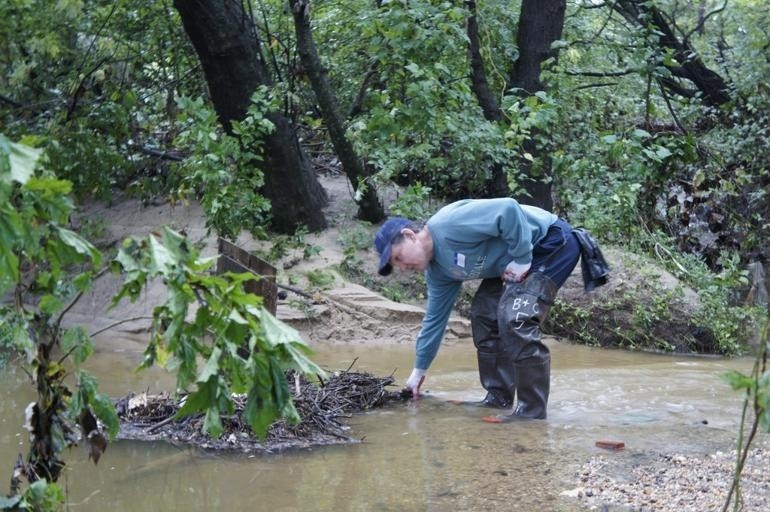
[
  {"x1": 403, "y1": 367, "x2": 427, "y2": 400},
  {"x1": 570, "y1": 228, "x2": 609, "y2": 290},
  {"x1": 502, "y1": 259, "x2": 532, "y2": 282}
]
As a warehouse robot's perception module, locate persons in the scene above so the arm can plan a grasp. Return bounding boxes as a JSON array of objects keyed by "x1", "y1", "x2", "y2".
[{"x1": 374, "y1": 197, "x2": 582, "y2": 424}]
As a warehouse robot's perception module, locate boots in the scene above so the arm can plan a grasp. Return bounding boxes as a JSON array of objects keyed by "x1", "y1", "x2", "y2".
[
  {"x1": 483, "y1": 359, "x2": 556, "y2": 423},
  {"x1": 447, "y1": 352, "x2": 516, "y2": 410}
]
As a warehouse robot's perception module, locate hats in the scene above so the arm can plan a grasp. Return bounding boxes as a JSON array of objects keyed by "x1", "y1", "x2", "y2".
[{"x1": 375, "y1": 217, "x2": 412, "y2": 275}]
